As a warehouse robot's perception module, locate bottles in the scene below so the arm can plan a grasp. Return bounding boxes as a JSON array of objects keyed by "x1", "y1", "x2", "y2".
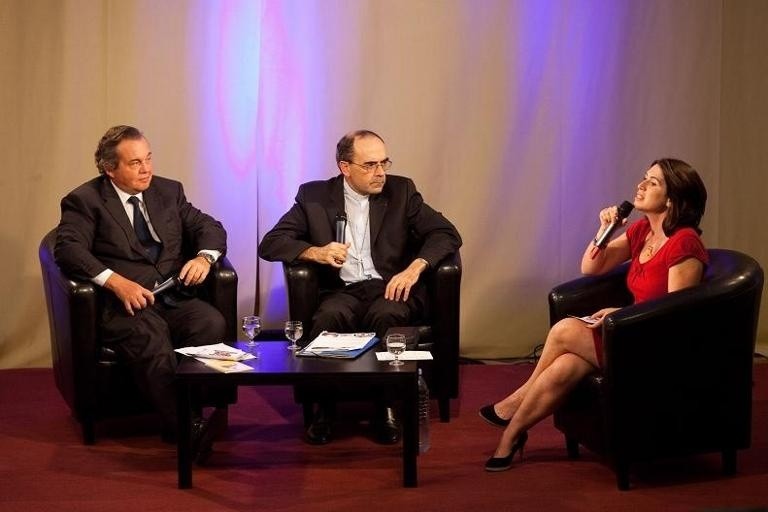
[{"x1": 417, "y1": 368, "x2": 430, "y2": 453}]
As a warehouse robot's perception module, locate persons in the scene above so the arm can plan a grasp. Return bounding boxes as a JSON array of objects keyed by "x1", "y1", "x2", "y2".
[
  {"x1": 256, "y1": 129, "x2": 464, "y2": 445},
  {"x1": 55, "y1": 124, "x2": 228, "y2": 446},
  {"x1": 477, "y1": 159, "x2": 710, "y2": 473}
]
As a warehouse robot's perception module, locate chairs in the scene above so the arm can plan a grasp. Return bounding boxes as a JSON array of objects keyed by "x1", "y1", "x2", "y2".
[{"x1": 549, "y1": 249, "x2": 764, "y2": 489}]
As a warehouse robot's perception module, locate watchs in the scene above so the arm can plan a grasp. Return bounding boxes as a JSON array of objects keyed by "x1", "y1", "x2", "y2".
[{"x1": 196, "y1": 252, "x2": 215, "y2": 265}]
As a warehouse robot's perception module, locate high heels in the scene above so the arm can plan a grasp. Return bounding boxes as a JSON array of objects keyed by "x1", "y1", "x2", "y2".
[
  {"x1": 479, "y1": 403, "x2": 512, "y2": 428},
  {"x1": 485, "y1": 431, "x2": 528, "y2": 471}
]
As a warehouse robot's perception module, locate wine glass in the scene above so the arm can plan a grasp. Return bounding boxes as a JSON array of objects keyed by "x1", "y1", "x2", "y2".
[
  {"x1": 241, "y1": 316, "x2": 262, "y2": 347},
  {"x1": 386, "y1": 332, "x2": 406, "y2": 365},
  {"x1": 284, "y1": 320, "x2": 304, "y2": 349}
]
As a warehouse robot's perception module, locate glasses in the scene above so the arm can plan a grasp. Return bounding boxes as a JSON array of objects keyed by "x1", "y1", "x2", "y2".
[{"x1": 347, "y1": 161, "x2": 392, "y2": 175}]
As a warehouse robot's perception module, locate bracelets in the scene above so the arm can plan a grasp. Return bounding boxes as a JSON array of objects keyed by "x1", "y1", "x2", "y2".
[{"x1": 593, "y1": 234, "x2": 610, "y2": 249}]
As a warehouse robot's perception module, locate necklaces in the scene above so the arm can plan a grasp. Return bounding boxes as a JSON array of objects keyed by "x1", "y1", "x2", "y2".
[{"x1": 645, "y1": 242, "x2": 656, "y2": 256}]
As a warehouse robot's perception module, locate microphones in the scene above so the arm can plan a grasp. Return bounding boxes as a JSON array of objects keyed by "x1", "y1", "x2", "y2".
[
  {"x1": 590, "y1": 200, "x2": 634, "y2": 259},
  {"x1": 335, "y1": 211, "x2": 348, "y2": 264},
  {"x1": 150, "y1": 274, "x2": 183, "y2": 296}
]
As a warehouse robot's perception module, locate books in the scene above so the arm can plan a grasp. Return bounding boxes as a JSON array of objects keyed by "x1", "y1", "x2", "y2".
[{"x1": 297, "y1": 335, "x2": 381, "y2": 360}]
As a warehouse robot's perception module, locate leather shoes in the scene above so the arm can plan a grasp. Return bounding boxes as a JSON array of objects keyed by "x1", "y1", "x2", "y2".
[
  {"x1": 191, "y1": 423, "x2": 206, "y2": 441},
  {"x1": 377, "y1": 407, "x2": 400, "y2": 443},
  {"x1": 306, "y1": 418, "x2": 333, "y2": 444}
]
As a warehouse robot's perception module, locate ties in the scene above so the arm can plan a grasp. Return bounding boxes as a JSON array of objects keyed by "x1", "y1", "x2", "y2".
[{"x1": 129, "y1": 196, "x2": 162, "y2": 260}]
